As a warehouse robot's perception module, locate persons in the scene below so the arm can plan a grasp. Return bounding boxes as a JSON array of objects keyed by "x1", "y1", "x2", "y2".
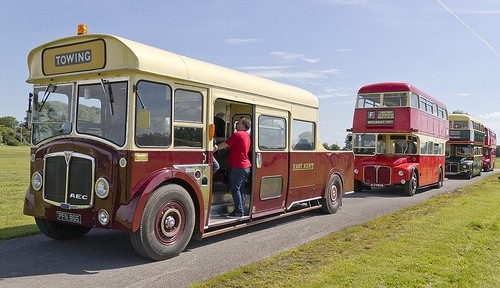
[
  {"x1": 213, "y1": 118, "x2": 251, "y2": 219},
  {"x1": 213, "y1": 112, "x2": 226, "y2": 175}
]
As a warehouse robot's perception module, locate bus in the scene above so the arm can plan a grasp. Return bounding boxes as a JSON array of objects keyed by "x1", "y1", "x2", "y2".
[
  {"x1": 351, "y1": 82, "x2": 450, "y2": 197},
  {"x1": 25, "y1": 24, "x2": 354, "y2": 262},
  {"x1": 483, "y1": 127, "x2": 496, "y2": 172},
  {"x1": 445, "y1": 114, "x2": 484, "y2": 179}
]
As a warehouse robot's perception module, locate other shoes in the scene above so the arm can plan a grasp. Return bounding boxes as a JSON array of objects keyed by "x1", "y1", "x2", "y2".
[{"x1": 226, "y1": 210, "x2": 244, "y2": 219}]
